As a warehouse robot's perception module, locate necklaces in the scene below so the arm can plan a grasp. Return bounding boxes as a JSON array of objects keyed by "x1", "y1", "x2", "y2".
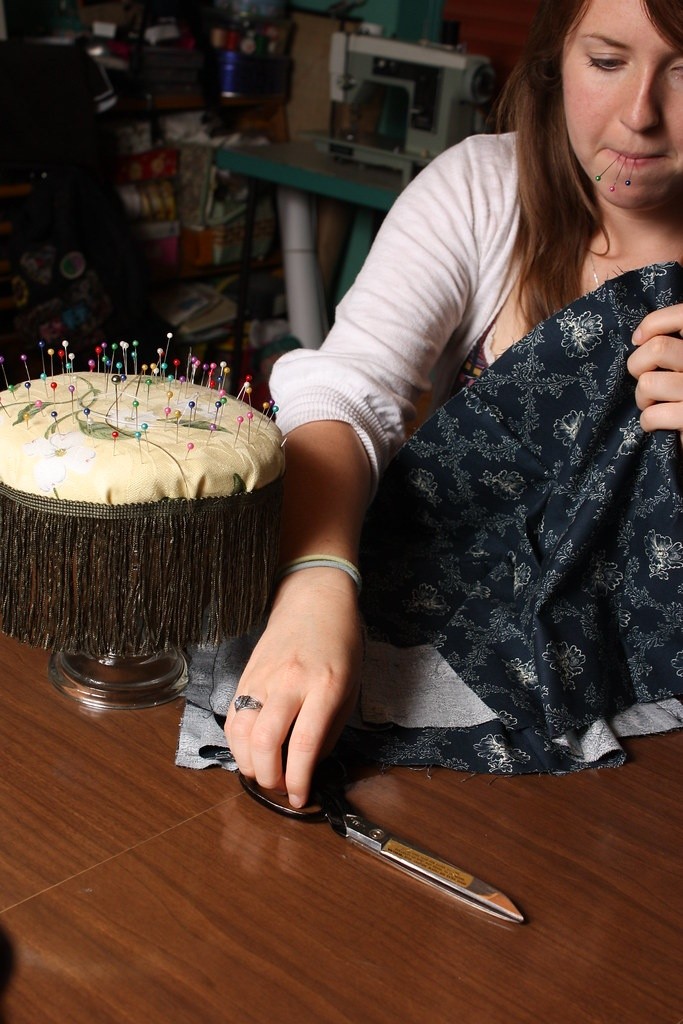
[{"x1": 587, "y1": 238, "x2": 623, "y2": 288}]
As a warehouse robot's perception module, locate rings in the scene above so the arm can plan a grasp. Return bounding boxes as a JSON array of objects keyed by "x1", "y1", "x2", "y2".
[{"x1": 235, "y1": 695, "x2": 263, "y2": 712}]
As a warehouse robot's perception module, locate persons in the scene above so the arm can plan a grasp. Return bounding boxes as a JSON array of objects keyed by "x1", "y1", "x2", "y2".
[{"x1": 223, "y1": 0, "x2": 683, "y2": 812}]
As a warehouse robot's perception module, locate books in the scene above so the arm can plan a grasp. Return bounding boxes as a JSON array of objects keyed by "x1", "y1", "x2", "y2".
[{"x1": 151, "y1": 282, "x2": 239, "y2": 343}]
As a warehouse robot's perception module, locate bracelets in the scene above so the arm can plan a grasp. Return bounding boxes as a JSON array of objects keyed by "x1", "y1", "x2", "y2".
[{"x1": 282, "y1": 553, "x2": 362, "y2": 593}]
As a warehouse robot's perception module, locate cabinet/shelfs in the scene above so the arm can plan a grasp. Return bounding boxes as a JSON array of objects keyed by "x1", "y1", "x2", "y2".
[{"x1": 95, "y1": 89, "x2": 292, "y2": 284}]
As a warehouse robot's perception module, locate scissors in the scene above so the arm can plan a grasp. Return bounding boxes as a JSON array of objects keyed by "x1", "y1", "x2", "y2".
[{"x1": 239, "y1": 755, "x2": 527, "y2": 924}]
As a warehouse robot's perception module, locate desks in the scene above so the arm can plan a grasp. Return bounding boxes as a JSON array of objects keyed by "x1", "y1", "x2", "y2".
[
  {"x1": 212, "y1": 133, "x2": 423, "y2": 397},
  {"x1": 0, "y1": 633, "x2": 682, "y2": 1021}
]
